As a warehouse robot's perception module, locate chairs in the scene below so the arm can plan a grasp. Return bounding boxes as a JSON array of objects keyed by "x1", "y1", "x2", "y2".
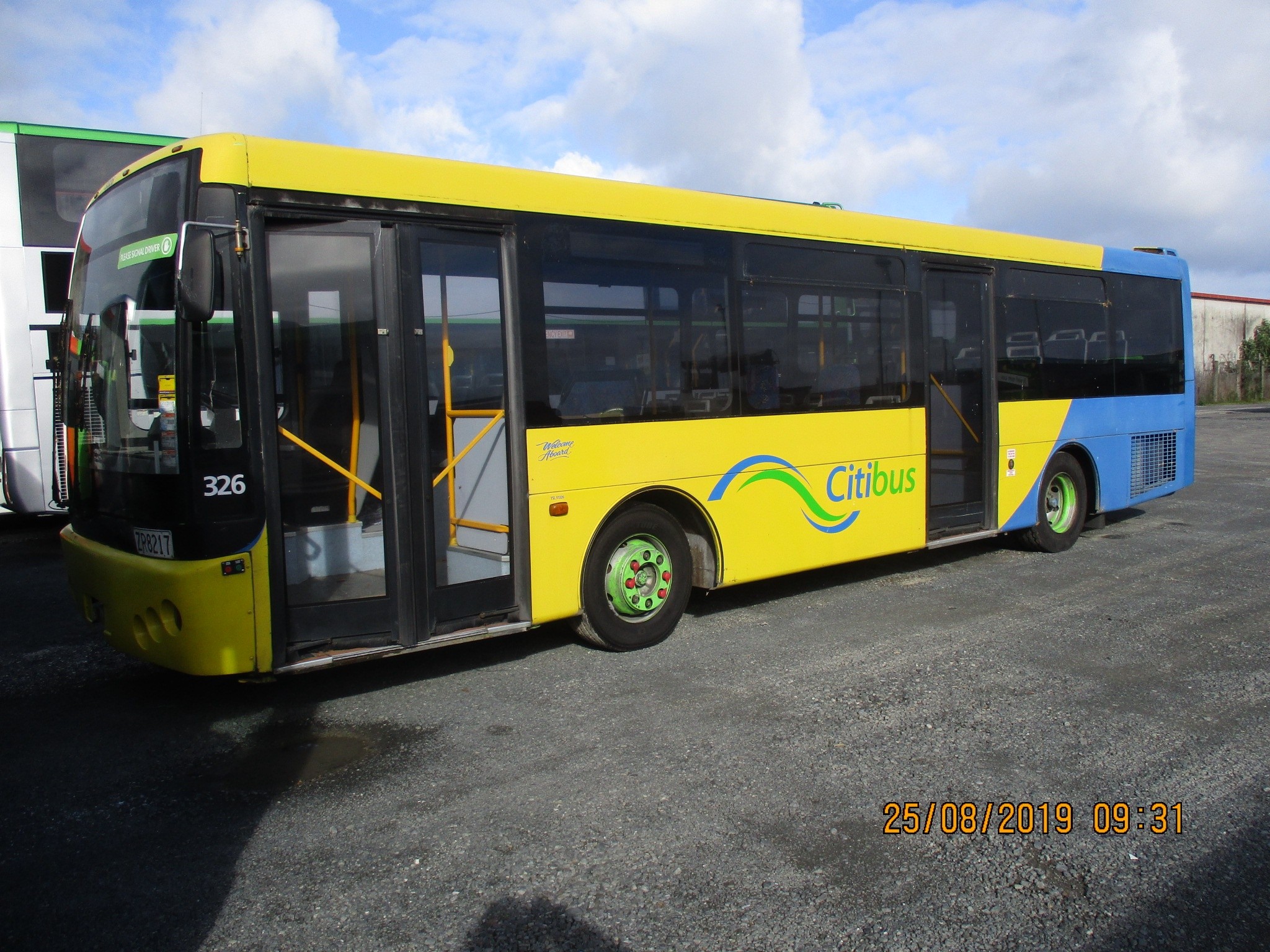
[
  {"x1": 1086, "y1": 329, "x2": 1129, "y2": 362},
  {"x1": 953, "y1": 347, "x2": 982, "y2": 370},
  {"x1": 474, "y1": 372, "x2": 506, "y2": 410},
  {"x1": 440, "y1": 375, "x2": 477, "y2": 412},
  {"x1": 1006, "y1": 331, "x2": 1042, "y2": 356},
  {"x1": 1042, "y1": 328, "x2": 1089, "y2": 364},
  {"x1": 1007, "y1": 344, "x2": 1044, "y2": 366}
]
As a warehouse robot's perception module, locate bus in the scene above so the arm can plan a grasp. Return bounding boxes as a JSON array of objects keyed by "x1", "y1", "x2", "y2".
[
  {"x1": 2, "y1": 117, "x2": 190, "y2": 521},
  {"x1": 51, "y1": 131, "x2": 1195, "y2": 688}
]
